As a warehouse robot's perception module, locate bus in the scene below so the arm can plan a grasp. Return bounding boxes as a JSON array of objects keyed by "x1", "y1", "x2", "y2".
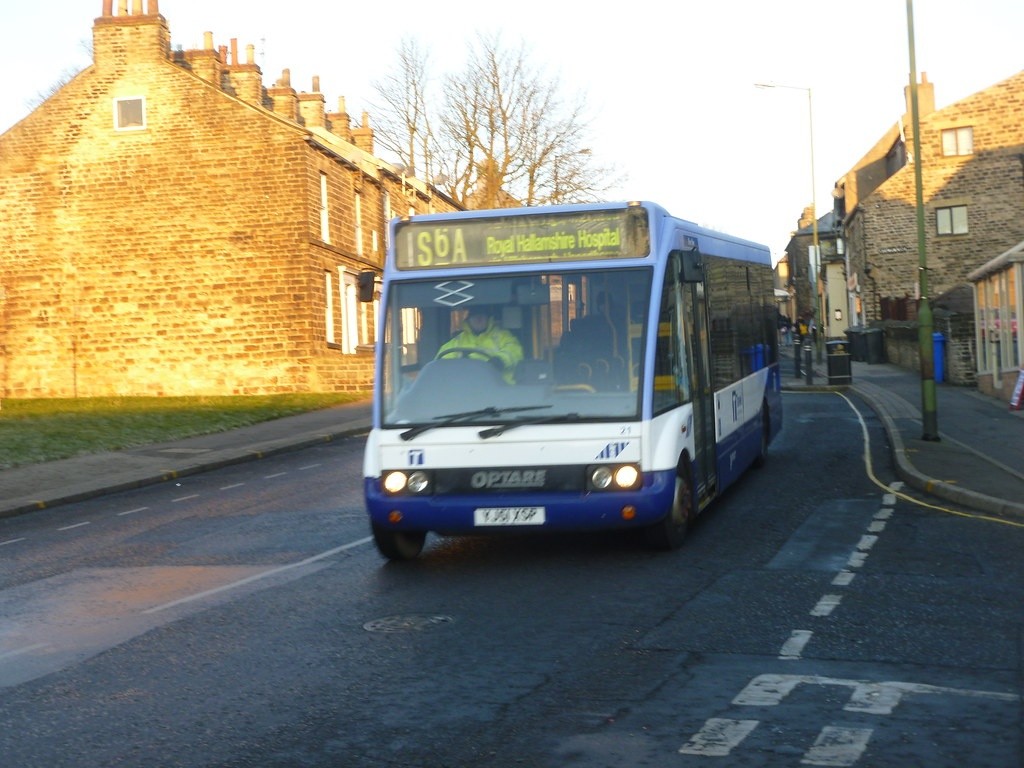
[{"x1": 354, "y1": 201, "x2": 776, "y2": 564}]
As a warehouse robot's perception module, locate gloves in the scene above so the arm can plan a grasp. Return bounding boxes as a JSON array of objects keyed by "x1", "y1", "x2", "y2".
[{"x1": 488, "y1": 355, "x2": 504, "y2": 370}]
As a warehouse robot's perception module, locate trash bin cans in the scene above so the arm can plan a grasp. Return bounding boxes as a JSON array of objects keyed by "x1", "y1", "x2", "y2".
[
  {"x1": 748, "y1": 344, "x2": 772, "y2": 372},
  {"x1": 931, "y1": 332, "x2": 944, "y2": 384},
  {"x1": 859, "y1": 328, "x2": 885, "y2": 364},
  {"x1": 826, "y1": 340, "x2": 853, "y2": 386},
  {"x1": 843, "y1": 326, "x2": 865, "y2": 362}
]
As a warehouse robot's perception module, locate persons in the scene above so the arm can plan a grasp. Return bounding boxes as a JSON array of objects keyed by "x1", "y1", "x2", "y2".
[
  {"x1": 597, "y1": 292, "x2": 621, "y2": 319},
  {"x1": 795, "y1": 316, "x2": 808, "y2": 347},
  {"x1": 808, "y1": 313, "x2": 816, "y2": 343},
  {"x1": 777, "y1": 314, "x2": 792, "y2": 346},
  {"x1": 437, "y1": 305, "x2": 525, "y2": 388}
]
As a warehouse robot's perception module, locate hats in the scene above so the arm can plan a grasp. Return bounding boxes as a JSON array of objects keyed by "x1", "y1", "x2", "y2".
[{"x1": 468, "y1": 306, "x2": 490, "y2": 316}]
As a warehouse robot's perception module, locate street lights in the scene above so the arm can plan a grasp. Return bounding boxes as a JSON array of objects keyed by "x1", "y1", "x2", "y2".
[{"x1": 755, "y1": 81, "x2": 823, "y2": 367}]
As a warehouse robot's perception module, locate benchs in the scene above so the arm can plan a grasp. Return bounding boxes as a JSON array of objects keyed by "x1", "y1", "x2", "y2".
[{"x1": 542, "y1": 314, "x2": 637, "y2": 394}]
